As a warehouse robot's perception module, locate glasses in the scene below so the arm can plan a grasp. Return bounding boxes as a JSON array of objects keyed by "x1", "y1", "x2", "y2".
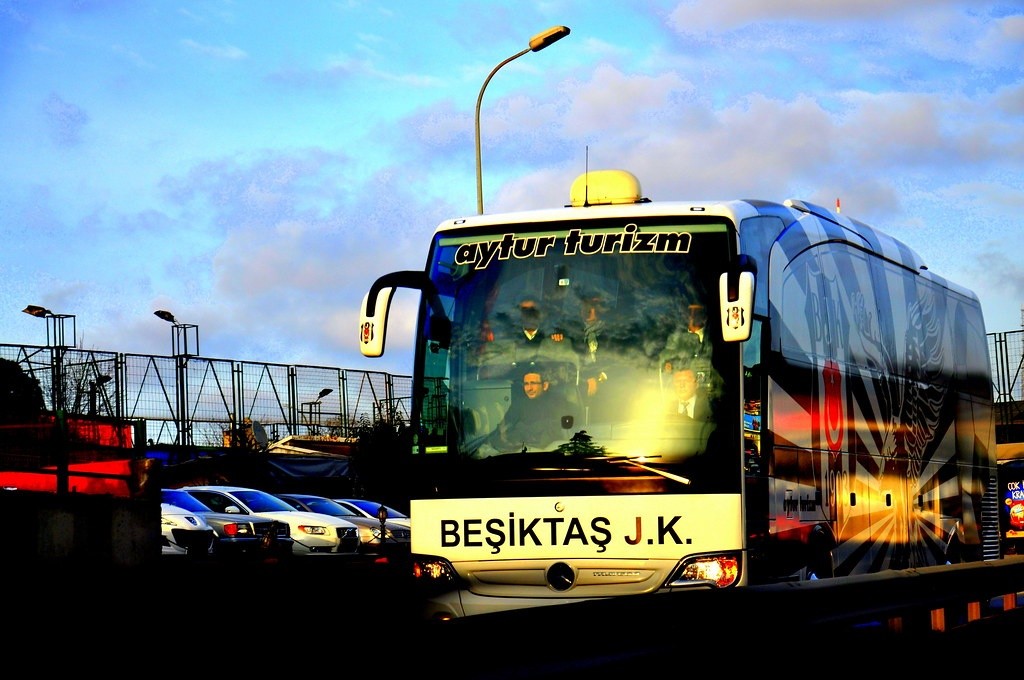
[{"x1": 522, "y1": 381, "x2": 544, "y2": 385}]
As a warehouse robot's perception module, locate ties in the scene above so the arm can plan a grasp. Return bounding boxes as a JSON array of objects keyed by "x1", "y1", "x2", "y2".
[{"x1": 681, "y1": 402, "x2": 689, "y2": 415}]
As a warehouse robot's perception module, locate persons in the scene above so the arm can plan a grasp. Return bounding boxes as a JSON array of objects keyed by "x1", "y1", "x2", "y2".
[
  {"x1": 505, "y1": 367, "x2": 567, "y2": 446},
  {"x1": 667, "y1": 369, "x2": 704, "y2": 419},
  {"x1": 496, "y1": 292, "x2": 714, "y2": 425}
]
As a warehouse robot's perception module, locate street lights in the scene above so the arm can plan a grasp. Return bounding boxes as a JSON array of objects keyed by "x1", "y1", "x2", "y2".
[{"x1": 474, "y1": 24, "x2": 574, "y2": 215}]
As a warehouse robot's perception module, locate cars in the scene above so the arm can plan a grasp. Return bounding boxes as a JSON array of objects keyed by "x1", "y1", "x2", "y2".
[
  {"x1": 159, "y1": 502, "x2": 218, "y2": 558},
  {"x1": 332, "y1": 498, "x2": 410, "y2": 529},
  {"x1": 178, "y1": 485, "x2": 363, "y2": 566},
  {"x1": 272, "y1": 494, "x2": 411, "y2": 561},
  {"x1": 159, "y1": 489, "x2": 294, "y2": 561}
]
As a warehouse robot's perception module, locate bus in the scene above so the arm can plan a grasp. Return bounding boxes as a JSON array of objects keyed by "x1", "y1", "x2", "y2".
[
  {"x1": 994, "y1": 400, "x2": 1024, "y2": 555},
  {"x1": 358, "y1": 172, "x2": 1006, "y2": 627}
]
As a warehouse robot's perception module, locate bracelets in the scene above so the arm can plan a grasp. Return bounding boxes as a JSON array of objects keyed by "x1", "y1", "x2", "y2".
[{"x1": 595, "y1": 375, "x2": 604, "y2": 384}]
